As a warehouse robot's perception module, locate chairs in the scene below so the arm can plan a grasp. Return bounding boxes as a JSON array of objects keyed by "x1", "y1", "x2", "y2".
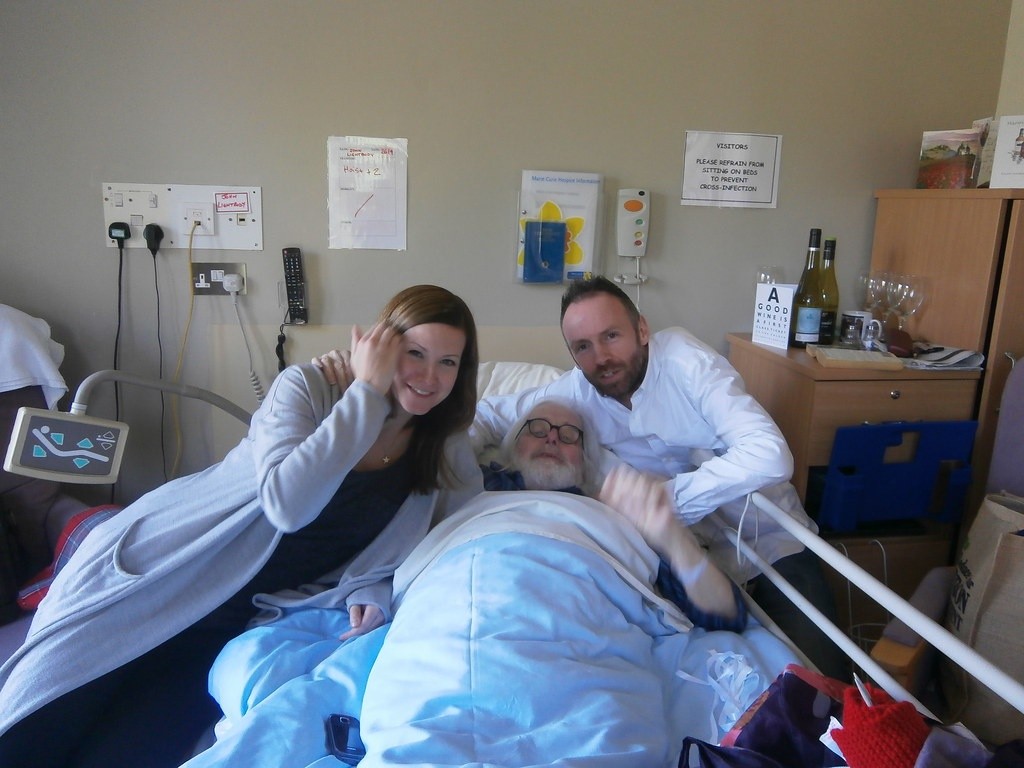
[{"x1": 0, "y1": 302, "x2": 129, "y2": 668}]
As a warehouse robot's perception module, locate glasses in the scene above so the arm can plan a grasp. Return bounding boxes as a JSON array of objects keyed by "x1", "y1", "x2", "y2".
[{"x1": 513, "y1": 418, "x2": 585, "y2": 452}]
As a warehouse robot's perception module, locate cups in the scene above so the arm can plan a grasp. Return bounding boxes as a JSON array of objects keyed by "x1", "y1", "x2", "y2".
[{"x1": 841, "y1": 310, "x2": 882, "y2": 348}]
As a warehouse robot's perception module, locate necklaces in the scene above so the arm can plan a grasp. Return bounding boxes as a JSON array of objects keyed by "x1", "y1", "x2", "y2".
[{"x1": 375, "y1": 432, "x2": 403, "y2": 465}]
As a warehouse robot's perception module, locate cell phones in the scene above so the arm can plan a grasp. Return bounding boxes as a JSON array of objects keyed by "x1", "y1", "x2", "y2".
[{"x1": 326, "y1": 713, "x2": 366, "y2": 766}]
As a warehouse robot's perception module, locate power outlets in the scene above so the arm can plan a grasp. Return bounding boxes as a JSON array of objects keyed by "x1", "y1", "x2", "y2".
[
  {"x1": 102, "y1": 182, "x2": 177, "y2": 249},
  {"x1": 192, "y1": 263, "x2": 246, "y2": 296},
  {"x1": 176, "y1": 184, "x2": 264, "y2": 250}
]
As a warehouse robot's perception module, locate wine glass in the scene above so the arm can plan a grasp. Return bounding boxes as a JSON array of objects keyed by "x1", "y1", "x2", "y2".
[{"x1": 859, "y1": 271, "x2": 925, "y2": 330}]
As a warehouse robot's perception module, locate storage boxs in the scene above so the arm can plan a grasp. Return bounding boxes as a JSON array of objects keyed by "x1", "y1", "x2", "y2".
[{"x1": 915, "y1": 127, "x2": 989, "y2": 189}]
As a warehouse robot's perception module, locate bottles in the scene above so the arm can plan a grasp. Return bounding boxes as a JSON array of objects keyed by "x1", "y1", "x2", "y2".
[
  {"x1": 821, "y1": 235, "x2": 838, "y2": 345},
  {"x1": 789, "y1": 228, "x2": 823, "y2": 347}
]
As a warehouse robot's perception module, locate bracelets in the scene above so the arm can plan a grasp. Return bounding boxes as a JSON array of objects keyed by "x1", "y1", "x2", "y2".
[{"x1": 677, "y1": 554, "x2": 708, "y2": 587}]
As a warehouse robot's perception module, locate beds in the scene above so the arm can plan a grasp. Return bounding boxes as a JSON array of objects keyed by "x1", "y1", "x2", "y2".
[{"x1": 192, "y1": 324, "x2": 1024, "y2": 768}]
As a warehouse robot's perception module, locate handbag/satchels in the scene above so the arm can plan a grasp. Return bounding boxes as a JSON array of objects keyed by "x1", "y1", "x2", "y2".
[{"x1": 944, "y1": 492, "x2": 1024, "y2": 743}]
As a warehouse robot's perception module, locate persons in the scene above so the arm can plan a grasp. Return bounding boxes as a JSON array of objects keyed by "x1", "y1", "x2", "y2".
[
  {"x1": 312, "y1": 273, "x2": 848, "y2": 680},
  {"x1": 0, "y1": 285, "x2": 479, "y2": 768},
  {"x1": 477, "y1": 395, "x2": 750, "y2": 632}
]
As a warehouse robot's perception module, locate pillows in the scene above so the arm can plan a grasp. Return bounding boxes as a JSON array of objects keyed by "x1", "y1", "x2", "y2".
[{"x1": 476, "y1": 362, "x2": 568, "y2": 399}]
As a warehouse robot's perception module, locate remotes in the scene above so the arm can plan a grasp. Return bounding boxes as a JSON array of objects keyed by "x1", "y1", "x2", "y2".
[{"x1": 282, "y1": 247, "x2": 311, "y2": 323}]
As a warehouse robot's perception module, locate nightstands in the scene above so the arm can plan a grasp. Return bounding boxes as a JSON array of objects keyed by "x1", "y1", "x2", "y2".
[{"x1": 726, "y1": 331, "x2": 982, "y2": 650}]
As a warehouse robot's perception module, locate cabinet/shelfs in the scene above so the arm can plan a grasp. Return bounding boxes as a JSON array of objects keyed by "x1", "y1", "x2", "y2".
[{"x1": 867, "y1": 186, "x2": 1024, "y2": 571}]
